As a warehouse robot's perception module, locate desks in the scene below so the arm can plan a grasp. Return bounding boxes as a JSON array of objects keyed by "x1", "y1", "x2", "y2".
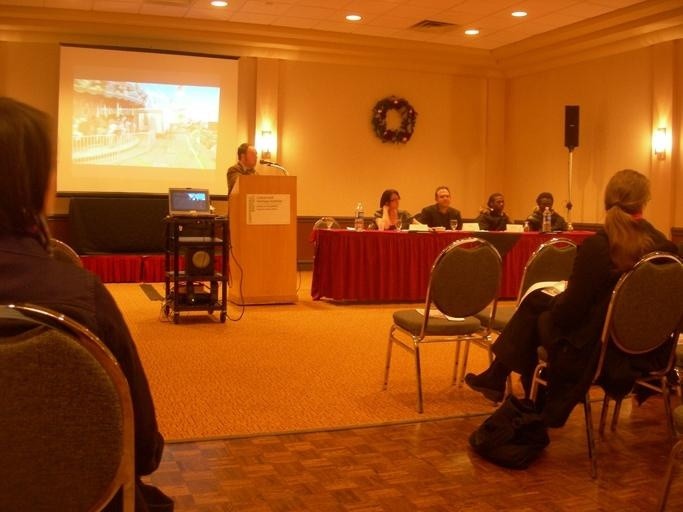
[{"x1": 311, "y1": 228, "x2": 599, "y2": 304}]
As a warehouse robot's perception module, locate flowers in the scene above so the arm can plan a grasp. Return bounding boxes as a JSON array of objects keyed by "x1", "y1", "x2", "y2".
[{"x1": 370, "y1": 96, "x2": 417, "y2": 144}]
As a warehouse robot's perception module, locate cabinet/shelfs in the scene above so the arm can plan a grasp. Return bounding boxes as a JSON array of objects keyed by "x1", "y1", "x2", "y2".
[{"x1": 162, "y1": 213, "x2": 230, "y2": 324}]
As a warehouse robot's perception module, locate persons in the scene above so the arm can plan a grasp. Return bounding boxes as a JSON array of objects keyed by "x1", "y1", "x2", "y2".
[
  {"x1": 465, "y1": 169, "x2": 678, "y2": 403},
  {"x1": 367, "y1": 190, "x2": 422, "y2": 234},
  {"x1": 2, "y1": 98, "x2": 174, "y2": 511},
  {"x1": 421, "y1": 187, "x2": 462, "y2": 230},
  {"x1": 526, "y1": 191, "x2": 569, "y2": 230},
  {"x1": 226, "y1": 145, "x2": 257, "y2": 188},
  {"x1": 479, "y1": 192, "x2": 512, "y2": 230}
]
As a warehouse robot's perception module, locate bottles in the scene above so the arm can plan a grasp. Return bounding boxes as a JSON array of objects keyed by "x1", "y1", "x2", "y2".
[
  {"x1": 354, "y1": 202, "x2": 364, "y2": 230},
  {"x1": 542, "y1": 207, "x2": 552, "y2": 231}
]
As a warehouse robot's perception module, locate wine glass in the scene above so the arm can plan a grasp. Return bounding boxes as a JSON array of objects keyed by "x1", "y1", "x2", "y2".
[
  {"x1": 450, "y1": 219, "x2": 458, "y2": 231},
  {"x1": 523, "y1": 221, "x2": 530, "y2": 232},
  {"x1": 395, "y1": 218, "x2": 402, "y2": 231}
]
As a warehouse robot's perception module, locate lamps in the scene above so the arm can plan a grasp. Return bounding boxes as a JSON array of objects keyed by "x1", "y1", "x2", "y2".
[
  {"x1": 262, "y1": 131, "x2": 272, "y2": 159},
  {"x1": 654, "y1": 128, "x2": 666, "y2": 160}
]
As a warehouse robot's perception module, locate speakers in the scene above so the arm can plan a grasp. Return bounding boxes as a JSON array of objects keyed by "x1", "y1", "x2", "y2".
[{"x1": 564, "y1": 103, "x2": 579, "y2": 148}]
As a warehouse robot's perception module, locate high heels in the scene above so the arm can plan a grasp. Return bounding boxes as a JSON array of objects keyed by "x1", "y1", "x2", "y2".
[{"x1": 464, "y1": 368, "x2": 506, "y2": 402}]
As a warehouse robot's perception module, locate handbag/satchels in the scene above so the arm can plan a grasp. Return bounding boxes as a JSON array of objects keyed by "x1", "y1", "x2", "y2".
[{"x1": 466, "y1": 380, "x2": 552, "y2": 470}]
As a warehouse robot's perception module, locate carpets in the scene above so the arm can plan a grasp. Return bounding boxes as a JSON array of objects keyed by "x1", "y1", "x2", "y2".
[{"x1": 101, "y1": 270, "x2": 636, "y2": 445}]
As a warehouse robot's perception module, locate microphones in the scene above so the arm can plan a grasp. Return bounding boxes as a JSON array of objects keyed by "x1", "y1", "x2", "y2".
[{"x1": 260, "y1": 159, "x2": 276, "y2": 165}]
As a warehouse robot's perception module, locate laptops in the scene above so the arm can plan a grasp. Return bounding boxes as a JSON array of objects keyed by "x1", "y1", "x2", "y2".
[{"x1": 168, "y1": 187, "x2": 216, "y2": 219}]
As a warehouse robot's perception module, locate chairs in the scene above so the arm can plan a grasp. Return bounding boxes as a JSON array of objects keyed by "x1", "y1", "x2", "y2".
[
  {"x1": 382, "y1": 237, "x2": 503, "y2": 413},
  {"x1": 457, "y1": 236, "x2": 579, "y2": 395},
  {"x1": 0, "y1": 300, "x2": 136, "y2": 512},
  {"x1": 658, "y1": 402, "x2": 683, "y2": 512},
  {"x1": 313, "y1": 216, "x2": 341, "y2": 229},
  {"x1": 528, "y1": 250, "x2": 683, "y2": 479}
]
